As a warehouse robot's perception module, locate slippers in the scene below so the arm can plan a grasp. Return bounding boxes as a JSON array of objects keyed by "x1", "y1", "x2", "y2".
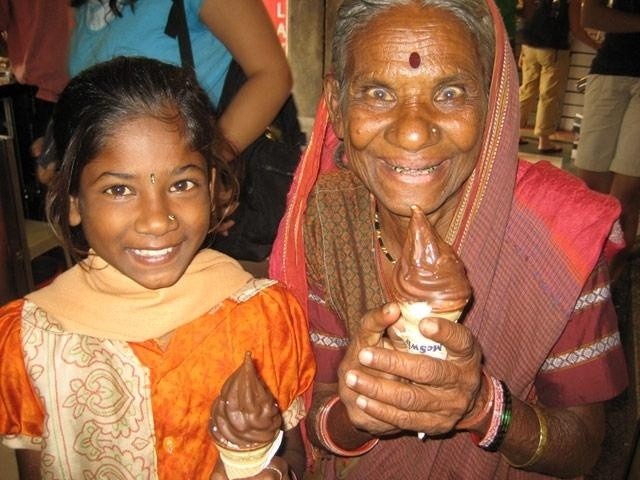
[{"x1": 519, "y1": 138, "x2": 563, "y2": 155}]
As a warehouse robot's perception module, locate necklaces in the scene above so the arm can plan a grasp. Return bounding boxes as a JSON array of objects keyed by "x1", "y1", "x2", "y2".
[{"x1": 373, "y1": 200, "x2": 398, "y2": 266}]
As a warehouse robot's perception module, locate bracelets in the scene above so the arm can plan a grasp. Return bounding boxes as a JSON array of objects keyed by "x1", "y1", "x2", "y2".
[
  {"x1": 314, "y1": 395, "x2": 381, "y2": 458},
  {"x1": 455, "y1": 364, "x2": 548, "y2": 468}
]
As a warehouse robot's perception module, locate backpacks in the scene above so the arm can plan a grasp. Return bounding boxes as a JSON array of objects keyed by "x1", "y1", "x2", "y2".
[{"x1": 163, "y1": 0, "x2": 308, "y2": 263}]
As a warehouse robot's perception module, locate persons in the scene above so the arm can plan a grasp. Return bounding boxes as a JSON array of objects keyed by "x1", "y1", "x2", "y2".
[
  {"x1": 514, "y1": 0, "x2": 604, "y2": 153},
  {"x1": 0, "y1": 0, "x2": 77, "y2": 141},
  {"x1": 0, "y1": 57, "x2": 318, "y2": 480},
  {"x1": 31, "y1": 0, "x2": 293, "y2": 259},
  {"x1": 269, "y1": 0, "x2": 629, "y2": 480},
  {"x1": 580, "y1": 0, "x2": 640, "y2": 287}
]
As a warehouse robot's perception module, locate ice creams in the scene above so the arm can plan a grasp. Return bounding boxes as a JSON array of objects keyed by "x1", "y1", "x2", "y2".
[
  {"x1": 385, "y1": 205, "x2": 472, "y2": 323},
  {"x1": 208, "y1": 350, "x2": 282, "y2": 480}
]
